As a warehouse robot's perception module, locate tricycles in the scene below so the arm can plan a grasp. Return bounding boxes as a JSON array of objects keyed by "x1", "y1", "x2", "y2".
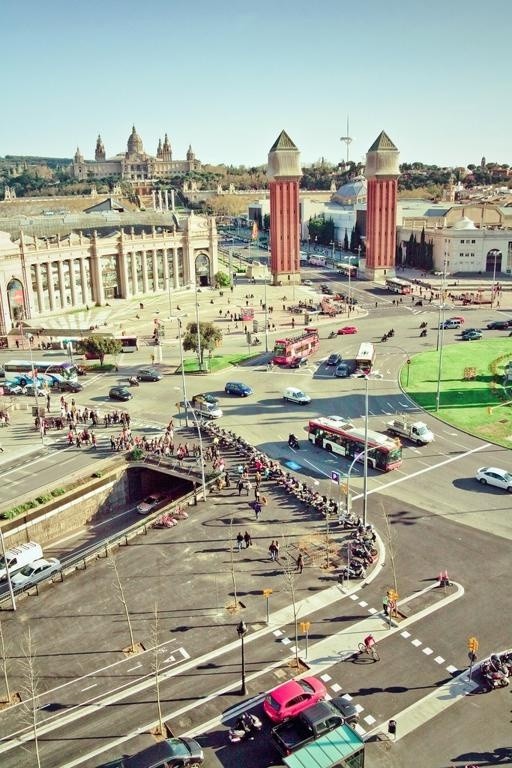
[{"x1": 282, "y1": 721, "x2": 365, "y2": 768}]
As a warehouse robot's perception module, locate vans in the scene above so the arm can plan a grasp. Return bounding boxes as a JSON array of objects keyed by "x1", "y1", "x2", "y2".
[
  {"x1": 283, "y1": 387, "x2": 312, "y2": 406},
  {"x1": 120, "y1": 737, "x2": 204, "y2": 768},
  {"x1": 0, "y1": 541, "x2": 44, "y2": 581}
]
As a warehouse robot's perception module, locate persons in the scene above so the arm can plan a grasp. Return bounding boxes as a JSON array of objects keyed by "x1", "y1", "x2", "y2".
[
  {"x1": 365, "y1": 634, "x2": 377, "y2": 655},
  {"x1": 383, "y1": 592, "x2": 391, "y2": 616},
  {"x1": 237, "y1": 533, "x2": 243, "y2": 552},
  {"x1": 275, "y1": 541, "x2": 279, "y2": 560},
  {"x1": 6, "y1": 243, "x2": 503, "y2": 519},
  {"x1": 295, "y1": 553, "x2": 304, "y2": 574},
  {"x1": 244, "y1": 712, "x2": 253, "y2": 727},
  {"x1": 269, "y1": 540, "x2": 277, "y2": 562},
  {"x1": 244, "y1": 531, "x2": 250, "y2": 548}
]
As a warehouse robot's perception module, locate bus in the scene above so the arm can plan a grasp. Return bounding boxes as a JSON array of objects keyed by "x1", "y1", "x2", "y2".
[
  {"x1": 355, "y1": 342, "x2": 376, "y2": 370},
  {"x1": 337, "y1": 264, "x2": 357, "y2": 276},
  {"x1": 272, "y1": 333, "x2": 320, "y2": 364},
  {"x1": 308, "y1": 255, "x2": 325, "y2": 266},
  {"x1": 106, "y1": 335, "x2": 139, "y2": 353},
  {"x1": 5, "y1": 360, "x2": 78, "y2": 389},
  {"x1": 386, "y1": 279, "x2": 412, "y2": 295},
  {"x1": 308, "y1": 417, "x2": 403, "y2": 473}
]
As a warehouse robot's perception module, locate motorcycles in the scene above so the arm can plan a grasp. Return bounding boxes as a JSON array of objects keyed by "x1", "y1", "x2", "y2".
[
  {"x1": 153, "y1": 508, "x2": 189, "y2": 528},
  {"x1": 224, "y1": 712, "x2": 262, "y2": 746},
  {"x1": 198, "y1": 421, "x2": 379, "y2": 580},
  {"x1": 128, "y1": 376, "x2": 141, "y2": 386},
  {"x1": 480, "y1": 652, "x2": 512, "y2": 692}
]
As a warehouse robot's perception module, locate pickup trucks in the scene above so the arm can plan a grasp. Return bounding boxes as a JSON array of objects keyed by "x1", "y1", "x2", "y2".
[{"x1": 270, "y1": 697, "x2": 360, "y2": 758}]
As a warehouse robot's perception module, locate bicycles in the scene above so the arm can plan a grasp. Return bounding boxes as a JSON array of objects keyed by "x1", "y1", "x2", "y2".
[{"x1": 358, "y1": 643, "x2": 380, "y2": 661}]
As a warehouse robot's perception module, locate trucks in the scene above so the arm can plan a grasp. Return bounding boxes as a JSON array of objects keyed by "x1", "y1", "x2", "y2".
[
  {"x1": 194, "y1": 401, "x2": 222, "y2": 420},
  {"x1": 386, "y1": 419, "x2": 434, "y2": 445}
]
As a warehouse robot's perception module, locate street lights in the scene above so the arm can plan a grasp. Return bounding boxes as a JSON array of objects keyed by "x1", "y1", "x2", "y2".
[
  {"x1": 489, "y1": 250, "x2": 502, "y2": 309},
  {"x1": 196, "y1": 289, "x2": 203, "y2": 360},
  {"x1": 26, "y1": 332, "x2": 44, "y2": 445},
  {"x1": 173, "y1": 387, "x2": 206, "y2": 502},
  {"x1": 434, "y1": 269, "x2": 455, "y2": 412},
  {"x1": 176, "y1": 313, "x2": 188, "y2": 428},
  {"x1": 346, "y1": 437, "x2": 400, "y2": 513},
  {"x1": 315, "y1": 239, "x2": 361, "y2": 267},
  {"x1": 236, "y1": 620, "x2": 248, "y2": 696}
]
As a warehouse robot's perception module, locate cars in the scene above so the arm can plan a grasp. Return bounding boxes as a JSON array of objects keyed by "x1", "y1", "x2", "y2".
[
  {"x1": 320, "y1": 284, "x2": 335, "y2": 295},
  {"x1": 109, "y1": 387, "x2": 131, "y2": 401},
  {"x1": 136, "y1": 492, "x2": 172, "y2": 515},
  {"x1": 475, "y1": 466, "x2": 512, "y2": 493},
  {"x1": 0, "y1": 365, "x2": 83, "y2": 396},
  {"x1": 264, "y1": 677, "x2": 327, "y2": 723},
  {"x1": 225, "y1": 381, "x2": 254, "y2": 397},
  {"x1": 136, "y1": 367, "x2": 163, "y2": 381},
  {"x1": 440, "y1": 316, "x2": 512, "y2": 341},
  {"x1": 336, "y1": 292, "x2": 357, "y2": 305},
  {"x1": 304, "y1": 280, "x2": 313, "y2": 286},
  {"x1": 338, "y1": 326, "x2": 358, "y2": 334},
  {"x1": 10, "y1": 558, "x2": 61, "y2": 588},
  {"x1": 327, "y1": 352, "x2": 351, "y2": 377},
  {"x1": 291, "y1": 357, "x2": 308, "y2": 368},
  {"x1": 193, "y1": 393, "x2": 219, "y2": 406}
]
